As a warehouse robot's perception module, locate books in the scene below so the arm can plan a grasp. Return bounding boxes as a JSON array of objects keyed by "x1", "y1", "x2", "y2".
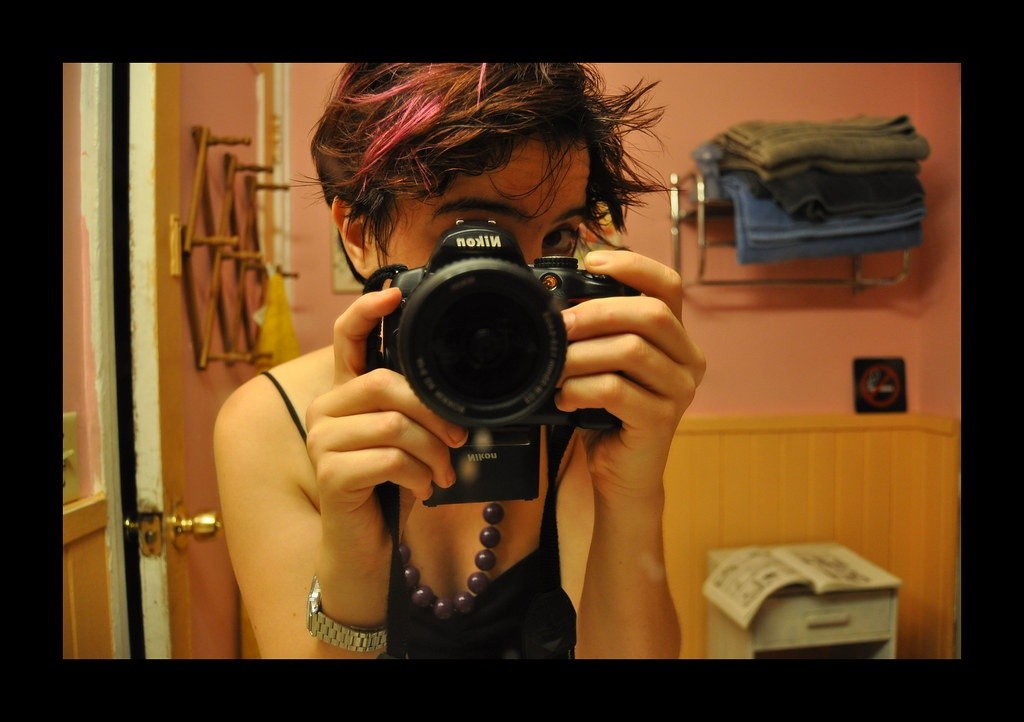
[{"x1": 700, "y1": 542, "x2": 903, "y2": 631}]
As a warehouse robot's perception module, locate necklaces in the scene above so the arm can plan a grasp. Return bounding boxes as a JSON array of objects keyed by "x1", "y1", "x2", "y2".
[{"x1": 397, "y1": 501, "x2": 503, "y2": 620}]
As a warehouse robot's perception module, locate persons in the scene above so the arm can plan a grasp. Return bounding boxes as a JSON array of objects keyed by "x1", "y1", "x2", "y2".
[{"x1": 208, "y1": 63, "x2": 707, "y2": 659}]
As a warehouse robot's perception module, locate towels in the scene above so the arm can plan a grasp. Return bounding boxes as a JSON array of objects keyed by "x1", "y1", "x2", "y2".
[
  {"x1": 691, "y1": 153, "x2": 924, "y2": 264},
  {"x1": 711, "y1": 113, "x2": 928, "y2": 179},
  {"x1": 745, "y1": 174, "x2": 925, "y2": 222}
]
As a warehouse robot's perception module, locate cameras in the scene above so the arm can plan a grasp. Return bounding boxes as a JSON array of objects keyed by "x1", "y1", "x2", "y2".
[{"x1": 381, "y1": 218, "x2": 640, "y2": 508}]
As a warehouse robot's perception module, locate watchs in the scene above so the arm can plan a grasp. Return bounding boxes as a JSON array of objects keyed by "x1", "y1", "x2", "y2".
[{"x1": 306, "y1": 574, "x2": 388, "y2": 652}]
